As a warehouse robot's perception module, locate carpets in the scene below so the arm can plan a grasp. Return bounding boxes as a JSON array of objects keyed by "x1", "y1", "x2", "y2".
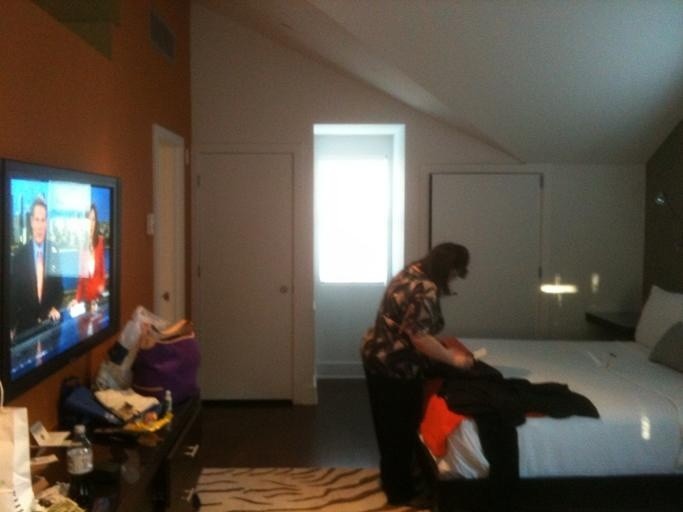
[{"x1": 193, "y1": 466, "x2": 431, "y2": 511}]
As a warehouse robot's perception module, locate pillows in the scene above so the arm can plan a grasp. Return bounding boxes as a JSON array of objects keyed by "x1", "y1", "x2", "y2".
[{"x1": 633, "y1": 285, "x2": 683, "y2": 374}]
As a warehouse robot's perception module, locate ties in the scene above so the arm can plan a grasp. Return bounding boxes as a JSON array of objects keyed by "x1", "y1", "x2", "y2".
[{"x1": 36, "y1": 249, "x2": 45, "y2": 304}]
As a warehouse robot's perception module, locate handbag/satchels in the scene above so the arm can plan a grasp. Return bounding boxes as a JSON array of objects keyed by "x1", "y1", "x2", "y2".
[{"x1": 130, "y1": 319, "x2": 200, "y2": 408}]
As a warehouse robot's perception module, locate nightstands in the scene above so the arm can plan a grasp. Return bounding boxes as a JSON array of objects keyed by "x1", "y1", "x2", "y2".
[{"x1": 584, "y1": 310, "x2": 641, "y2": 342}]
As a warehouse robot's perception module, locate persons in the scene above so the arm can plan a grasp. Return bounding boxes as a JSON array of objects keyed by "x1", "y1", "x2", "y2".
[
  {"x1": 11, "y1": 198, "x2": 65, "y2": 335},
  {"x1": 75, "y1": 203, "x2": 106, "y2": 309},
  {"x1": 359, "y1": 242, "x2": 475, "y2": 507}
]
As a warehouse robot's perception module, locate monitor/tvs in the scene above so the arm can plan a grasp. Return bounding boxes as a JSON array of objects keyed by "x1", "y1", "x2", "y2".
[{"x1": 0, "y1": 158, "x2": 120, "y2": 406}]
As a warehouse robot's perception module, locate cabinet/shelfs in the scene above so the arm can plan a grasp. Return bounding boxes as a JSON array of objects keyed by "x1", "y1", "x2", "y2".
[{"x1": 54, "y1": 390, "x2": 205, "y2": 511}]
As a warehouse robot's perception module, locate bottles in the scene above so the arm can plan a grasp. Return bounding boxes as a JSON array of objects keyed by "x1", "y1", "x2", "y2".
[{"x1": 66, "y1": 423, "x2": 97, "y2": 511}]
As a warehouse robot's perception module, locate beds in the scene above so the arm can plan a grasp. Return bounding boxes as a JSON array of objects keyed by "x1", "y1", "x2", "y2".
[{"x1": 429, "y1": 285, "x2": 682, "y2": 512}]
